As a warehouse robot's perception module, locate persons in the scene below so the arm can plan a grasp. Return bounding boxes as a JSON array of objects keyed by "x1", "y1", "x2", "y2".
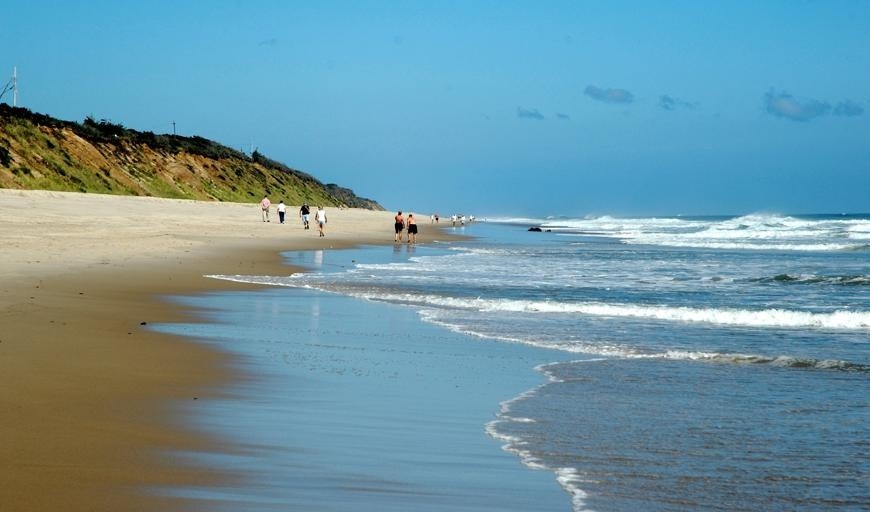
[
  {"x1": 261, "y1": 195, "x2": 326, "y2": 237},
  {"x1": 448, "y1": 213, "x2": 474, "y2": 228},
  {"x1": 430, "y1": 214, "x2": 439, "y2": 225},
  {"x1": 394, "y1": 210, "x2": 417, "y2": 243}
]
